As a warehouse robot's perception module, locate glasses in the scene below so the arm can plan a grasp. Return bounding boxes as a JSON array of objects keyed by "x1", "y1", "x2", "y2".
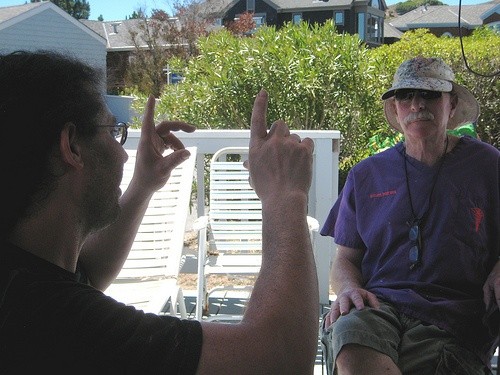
[
  {"x1": 394, "y1": 89, "x2": 442, "y2": 101},
  {"x1": 408, "y1": 222, "x2": 422, "y2": 271},
  {"x1": 96, "y1": 122, "x2": 129, "y2": 145}
]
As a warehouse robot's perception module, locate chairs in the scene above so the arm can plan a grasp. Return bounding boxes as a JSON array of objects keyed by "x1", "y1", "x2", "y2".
[{"x1": 103, "y1": 147, "x2": 320, "y2": 323}]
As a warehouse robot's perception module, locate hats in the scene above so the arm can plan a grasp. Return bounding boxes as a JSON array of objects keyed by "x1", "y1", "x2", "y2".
[{"x1": 382, "y1": 56, "x2": 480, "y2": 134}]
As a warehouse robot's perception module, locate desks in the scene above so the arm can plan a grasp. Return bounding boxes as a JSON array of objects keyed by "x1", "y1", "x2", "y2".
[{"x1": 113, "y1": 130, "x2": 341, "y2": 305}]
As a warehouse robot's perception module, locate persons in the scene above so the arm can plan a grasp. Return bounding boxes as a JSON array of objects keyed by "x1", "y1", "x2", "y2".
[
  {"x1": 0, "y1": 48, "x2": 321, "y2": 374},
  {"x1": 319, "y1": 55, "x2": 500, "y2": 374}
]
